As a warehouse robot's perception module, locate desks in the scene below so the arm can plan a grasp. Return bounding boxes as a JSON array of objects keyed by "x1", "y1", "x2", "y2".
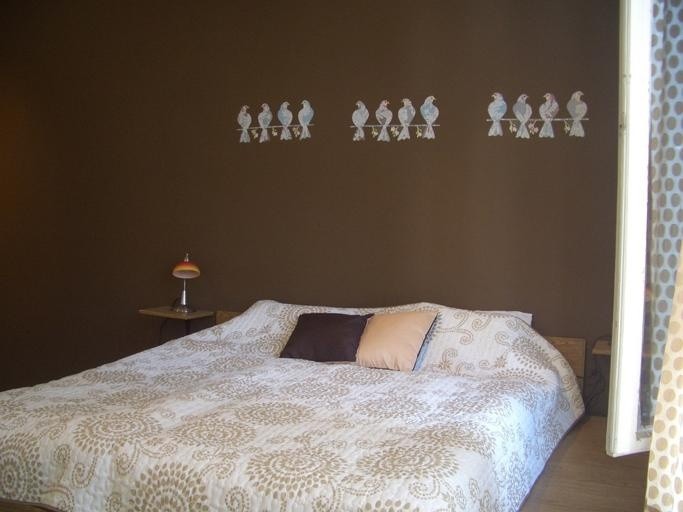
[
  {"x1": 139, "y1": 305, "x2": 214, "y2": 344},
  {"x1": 594, "y1": 337, "x2": 650, "y2": 360}
]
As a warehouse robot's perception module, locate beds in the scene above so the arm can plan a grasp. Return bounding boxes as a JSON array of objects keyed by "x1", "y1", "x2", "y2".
[{"x1": 0, "y1": 311, "x2": 586, "y2": 512}]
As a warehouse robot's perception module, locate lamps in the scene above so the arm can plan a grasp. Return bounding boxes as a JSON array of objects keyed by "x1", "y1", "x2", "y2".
[{"x1": 172, "y1": 253, "x2": 201, "y2": 312}]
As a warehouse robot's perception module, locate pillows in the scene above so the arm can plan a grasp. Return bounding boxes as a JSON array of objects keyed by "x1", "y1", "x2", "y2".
[
  {"x1": 356, "y1": 311, "x2": 438, "y2": 371},
  {"x1": 279, "y1": 314, "x2": 374, "y2": 362}
]
